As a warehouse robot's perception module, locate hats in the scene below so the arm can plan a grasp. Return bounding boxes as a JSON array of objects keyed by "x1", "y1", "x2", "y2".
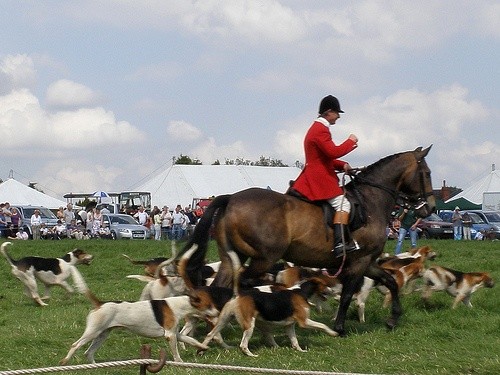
[
  {"x1": 177, "y1": 205, "x2": 182, "y2": 209},
  {"x1": 162, "y1": 206, "x2": 168, "y2": 209},
  {"x1": 19, "y1": 227, "x2": 24, "y2": 230},
  {"x1": 77, "y1": 220, "x2": 82, "y2": 224},
  {"x1": 154, "y1": 206, "x2": 157, "y2": 209},
  {"x1": 57, "y1": 220, "x2": 62, "y2": 223},
  {"x1": 455, "y1": 206, "x2": 460, "y2": 210}
]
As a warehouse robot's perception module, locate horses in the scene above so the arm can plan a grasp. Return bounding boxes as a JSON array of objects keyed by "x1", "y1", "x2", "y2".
[{"x1": 175, "y1": 144, "x2": 437, "y2": 337}]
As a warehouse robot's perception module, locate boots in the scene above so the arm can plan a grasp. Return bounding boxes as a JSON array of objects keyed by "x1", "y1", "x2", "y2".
[{"x1": 332, "y1": 223, "x2": 357, "y2": 258}]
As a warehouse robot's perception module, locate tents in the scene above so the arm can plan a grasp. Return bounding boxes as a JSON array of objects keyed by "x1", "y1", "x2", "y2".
[
  {"x1": 0, "y1": 179, "x2": 85, "y2": 209},
  {"x1": 129, "y1": 165, "x2": 354, "y2": 213}
]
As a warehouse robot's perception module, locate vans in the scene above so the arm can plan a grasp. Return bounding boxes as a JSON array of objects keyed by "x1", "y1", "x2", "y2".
[{"x1": 9, "y1": 205, "x2": 68, "y2": 239}]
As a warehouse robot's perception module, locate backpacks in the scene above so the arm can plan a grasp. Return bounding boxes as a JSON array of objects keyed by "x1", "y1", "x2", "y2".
[{"x1": 474, "y1": 231, "x2": 484, "y2": 240}]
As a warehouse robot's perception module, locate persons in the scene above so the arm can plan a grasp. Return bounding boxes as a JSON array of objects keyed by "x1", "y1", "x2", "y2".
[
  {"x1": 395, "y1": 206, "x2": 422, "y2": 253},
  {"x1": 31, "y1": 209, "x2": 42, "y2": 240},
  {"x1": 291, "y1": 95, "x2": 358, "y2": 258},
  {"x1": 132, "y1": 204, "x2": 191, "y2": 240},
  {"x1": 452, "y1": 206, "x2": 462, "y2": 240},
  {"x1": 0, "y1": 203, "x2": 19, "y2": 236},
  {"x1": 56, "y1": 203, "x2": 75, "y2": 224},
  {"x1": 196, "y1": 195, "x2": 215, "y2": 214},
  {"x1": 66, "y1": 219, "x2": 78, "y2": 239},
  {"x1": 40, "y1": 220, "x2": 66, "y2": 240},
  {"x1": 77, "y1": 205, "x2": 117, "y2": 240},
  {"x1": 461, "y1": 212, "x2": 472, "y2": 240}
]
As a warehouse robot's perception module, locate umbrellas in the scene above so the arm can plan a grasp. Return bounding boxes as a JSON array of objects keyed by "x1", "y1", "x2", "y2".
[
  {"x1": 444, "y1": 168, "x2": 500, "y2": 205},
  {"x1": 88, "y1": 191, "x2": 111, "y2": 198}
]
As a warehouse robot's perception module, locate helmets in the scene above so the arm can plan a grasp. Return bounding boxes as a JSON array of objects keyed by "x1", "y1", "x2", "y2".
[{"x1": 320, "y1": 95, "x2": 344, "y2": 113}]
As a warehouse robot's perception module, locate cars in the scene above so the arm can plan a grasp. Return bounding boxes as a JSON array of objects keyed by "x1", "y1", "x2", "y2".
[
  {"x1": 103, "y1": 214, "x2": 152, "y2": 240},
  {"x1": 389, "y1": 210, "x2": 500, "y2": 240}
]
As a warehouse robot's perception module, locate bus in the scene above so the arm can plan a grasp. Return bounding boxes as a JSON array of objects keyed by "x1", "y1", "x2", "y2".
[{"x1": 63, "y1": 191, "x2": 152, "y2": 216}]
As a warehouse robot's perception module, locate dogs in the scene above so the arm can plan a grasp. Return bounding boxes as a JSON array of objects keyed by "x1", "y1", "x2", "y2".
[
  {"x1": 57, "y1": 265, "x2": 220, "y2": 368},
  {"x1": 421, "y1": 264, "x2": 495, "y2": 309},
  {"x1": 0, "y1": 242, "x2": 96, "y2": 307},
  {"x1": 196, "y1": 249, "x2": 339, "y2": 357},
  {"x1": 121, "y1": 241, "x2": 436, "y2": 348}
]
